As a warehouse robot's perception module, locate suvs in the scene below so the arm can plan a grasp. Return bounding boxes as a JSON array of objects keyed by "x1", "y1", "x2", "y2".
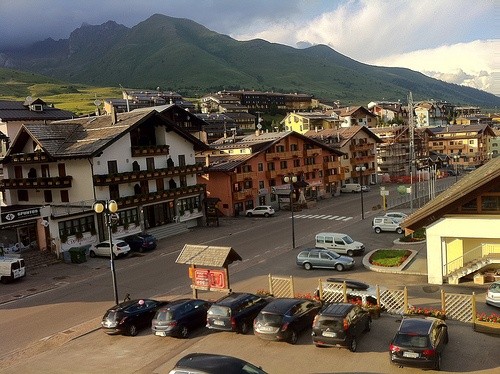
[
  {"x1": 206, "y1": 292, "x2": 273, "y2": 335},
  {"x1": 311, "y1": 301, "x2": 372, "y2": 352},
  {"x1": 246, "y1": 205, "x2": 276, "y2": 218}
]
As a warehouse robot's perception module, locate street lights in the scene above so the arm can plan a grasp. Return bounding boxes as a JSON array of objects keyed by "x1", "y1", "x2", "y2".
[
  {"x1": 454, "y1": 154, "x2": 460, "y2": 183},
  {"x1": 355, "y1": 165, "x2": 366, "y2": 219},
  {"x1": 283, "y1": 174, "x2": 298, "y2": 248},
  {"x1": 93, "y1": 200, "x2": 119, "y2": 305}
]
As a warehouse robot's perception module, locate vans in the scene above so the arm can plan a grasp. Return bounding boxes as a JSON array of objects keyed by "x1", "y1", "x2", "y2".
[
  {"x1": 0, "y1": 258, "x2": 27, "y2": 284},
  {"x1": 371, "y1": 216, "x2": 404, "y2": 235},
  {"x1": 315, "y1": 232, "x2": 365, "y2": 257},
  {"x1": 340, "y1": 184, "x2": 361, "y2": 193}
]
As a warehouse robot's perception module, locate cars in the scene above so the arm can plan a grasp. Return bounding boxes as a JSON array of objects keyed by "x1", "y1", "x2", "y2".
[
  {"x1": 362, "y1": 185, "x2": 369, "y2": 192},
  {"x1": 484, "y1": 280, "x2": 500, "y2": 308},
  {"x1": 101, "y1": 298, "x2": 163, "y2": 336},
  {"x1": 152, "y1": 298, "x2": 212, "y2": 339},
  {"x1": 123, "y1": 233, "x2": 158, "y2": 253},
  {"x1": 316, "y1": 278, "x2": 390, "y2": 306},
  {"x1": 296, "y1": 248, "x2": 355, "y2": 272},
  {"x1": 389, "y1": 314, "x2": 448, "y2": 371},
  {"x1": 168, "y1": 352, "x2": 268, "y2": 374},
  {"x1": 385, "y1": 212, "x2": 408, "y2": 219},
  {"x1": 89, "y1": 240, "x2": 131, "y2": 260},
  {"x1": 253, "y1": 298, "x2": 321, "y2": 345}
]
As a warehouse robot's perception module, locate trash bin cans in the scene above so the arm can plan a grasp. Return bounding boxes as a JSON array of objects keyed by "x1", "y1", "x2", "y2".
[{"x1": 68, "y1": 246, "x2": 86, "y2": 264}]
